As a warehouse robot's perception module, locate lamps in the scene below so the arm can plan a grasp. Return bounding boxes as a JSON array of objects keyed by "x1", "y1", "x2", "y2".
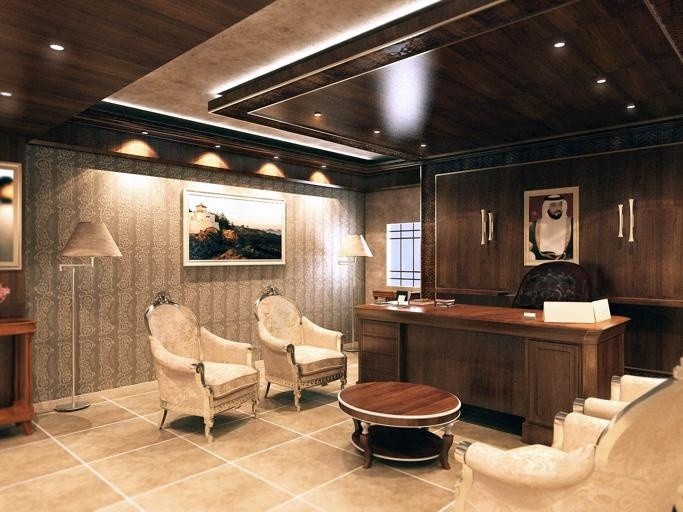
[
  {"x1": 54, "y1": 219, "x2": 123, "y2": 412},
  {"x1": 336, "y1": 232, "x2": 373, "y2": 353}
]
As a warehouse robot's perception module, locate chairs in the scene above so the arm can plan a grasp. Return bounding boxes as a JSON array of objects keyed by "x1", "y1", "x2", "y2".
[{"x1": 511, "y1": 260, "x2": 591, "y2": 310}]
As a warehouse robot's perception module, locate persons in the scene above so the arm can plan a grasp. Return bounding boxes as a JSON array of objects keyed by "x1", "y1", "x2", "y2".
[{"x1": 528, "y1": 193, "x2": 573, "y2": 260}]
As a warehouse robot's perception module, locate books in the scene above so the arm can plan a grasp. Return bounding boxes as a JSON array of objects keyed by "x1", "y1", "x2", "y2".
[{"x1": 409, "y1": 297, "x2": 456, "y2": 308}]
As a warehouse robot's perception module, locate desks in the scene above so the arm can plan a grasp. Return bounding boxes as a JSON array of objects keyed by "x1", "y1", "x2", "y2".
[{"x1": 353, "y1": 295, "x2": 632, "y2": 448}]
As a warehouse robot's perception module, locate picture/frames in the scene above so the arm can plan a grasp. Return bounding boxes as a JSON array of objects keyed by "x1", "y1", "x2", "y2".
[
  {"x1": 0, "y1": 161, "x2": 24, "y2": 272},
  {"x1": 522, "y1": 187, "x2": 580, "y2": 267},
  {"x1": 178, "y1": 188, "x2": 288, "y2": 269}
]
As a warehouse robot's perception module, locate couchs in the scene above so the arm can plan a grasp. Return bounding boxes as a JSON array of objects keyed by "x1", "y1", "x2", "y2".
[
  {"x1": 251, "y1": 284, "x2": 347, "y2": 411},
  {"x1": 443, "y1": 369, "x2": 682, "y2": 510},
  {"x1": 570, "y1": 370, "x2": 673, "y2": 424},
  {"x1": 142, "y1": 288, "x2": 264, "y2": 441}
]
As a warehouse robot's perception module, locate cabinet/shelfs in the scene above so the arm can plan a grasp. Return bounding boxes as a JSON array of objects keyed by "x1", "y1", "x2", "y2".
[
  {"x1": 0, "y1": 317, "x2": 36, "y2": 435},
  {"x1": 432, "y1": 170, "x2": 525, "y2": 297},
  {"x1": 579, "y1": 143, "x2": 683, "y2": 310}
]
